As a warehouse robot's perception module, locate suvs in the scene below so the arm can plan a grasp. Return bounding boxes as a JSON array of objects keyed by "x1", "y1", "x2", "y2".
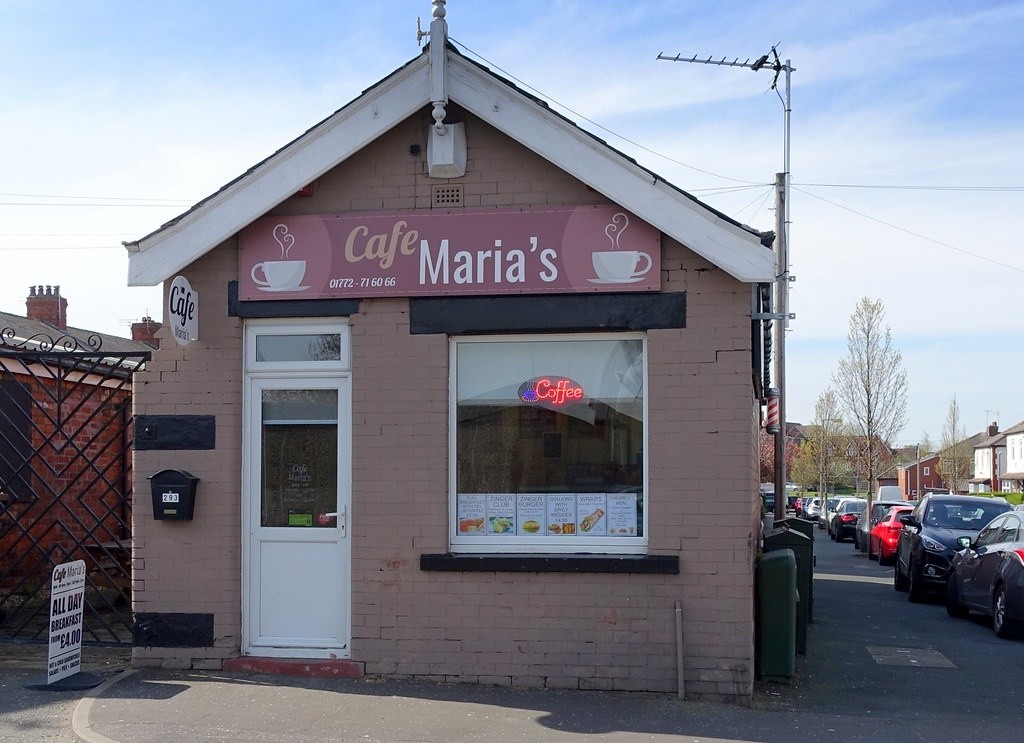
[{"x1": 894, "y1": 492, "x2": 1014, "y2": 603}]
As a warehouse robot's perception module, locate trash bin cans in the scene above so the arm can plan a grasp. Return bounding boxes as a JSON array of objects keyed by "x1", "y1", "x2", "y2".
[
  {"x1": 763, "y1": 517, "x2": 815, "y2": 653},
  {"x1": 759, "y1": 549, "x2": 798, "y2": 682}
]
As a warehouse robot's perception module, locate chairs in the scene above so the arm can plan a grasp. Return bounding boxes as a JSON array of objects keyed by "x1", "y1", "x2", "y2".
[
  {"x1": 933, "y1": 505, "x2": 950, "y2": 525},
  {"x1": 979, "y1": 512, "x2": 994, "y2": 524}
]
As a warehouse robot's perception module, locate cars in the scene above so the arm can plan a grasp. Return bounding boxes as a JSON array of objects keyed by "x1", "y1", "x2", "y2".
[
  {"x1": 942, "y1": 511, "x2": 1024, "y2": 638},
  {"x1": 829, "y1": 500, "x2": 870, "y2": 542},
  {"x1": 855, "y1": 501, "x2": 916, "y2": 554},
  {"x1": 807, "y1": 497, "x2": 823, "y2": 522},
  {"x1": 870, "y1": 506, "x2": 917, "y2": 565},
  {"x1": 762, "y1": 492, "x2": 813, "y2": 520},
  {"x1": 818, "y1": 497, "x2": 840, "y2": 530}
]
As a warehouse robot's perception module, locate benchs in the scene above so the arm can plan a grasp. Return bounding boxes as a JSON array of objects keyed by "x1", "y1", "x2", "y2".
[{"x1": 88, "y1": 561, "x2": 128, "y2": 572}]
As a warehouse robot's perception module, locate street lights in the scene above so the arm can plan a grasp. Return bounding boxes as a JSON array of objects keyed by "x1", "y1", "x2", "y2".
[
  {"x1": 904, "y1": 443, "x2": 921, "y2": 503},
  {"x1": 821, "y1": 418, "x2": 843, "y2": 532}
]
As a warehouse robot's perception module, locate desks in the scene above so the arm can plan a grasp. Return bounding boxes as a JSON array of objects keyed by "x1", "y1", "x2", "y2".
[{"x1": 86, "y1": 539, "x2": 132, "y2": 580}]
